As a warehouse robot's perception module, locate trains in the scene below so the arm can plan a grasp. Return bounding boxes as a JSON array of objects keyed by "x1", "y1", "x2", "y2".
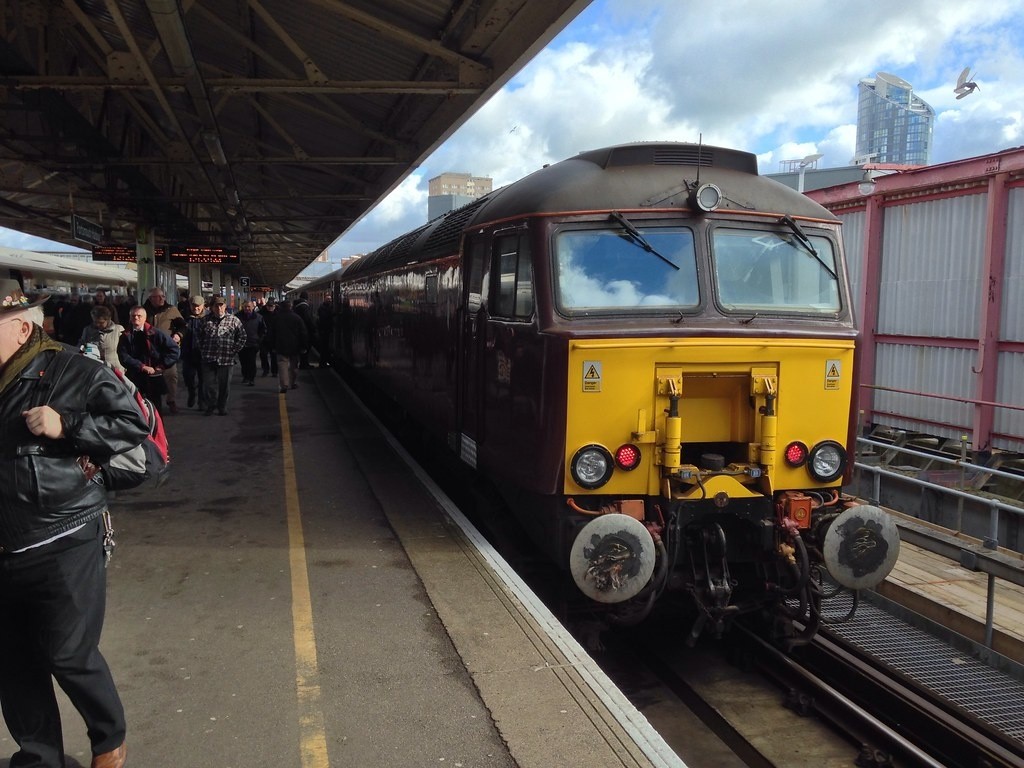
[
  {"x1": 283, "y1": 133, "x2": 901, "y2": 655},
  {"x1": 796, "y1": 146, "x2": 1024, "y2": 475}
]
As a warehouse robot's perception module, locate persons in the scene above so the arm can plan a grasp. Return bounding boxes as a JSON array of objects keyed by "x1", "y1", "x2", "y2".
[
  {"x1": 0, "y1": 288, "x2": 151, "y2": 768},
  {"x1": 53, "y1": 286, "x2": 331, "y2": 423}
]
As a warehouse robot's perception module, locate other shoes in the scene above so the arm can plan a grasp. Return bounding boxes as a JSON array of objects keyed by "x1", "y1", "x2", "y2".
[
  {"x1": 187, "y1": 390, "x2": 196, "y2": 408},
  {"x1": 218, "y1": 407, "x2": 227, "y2": 415},
  {"x1": 89, "y1": 739, "x2": 127, "y2": 768},
  {"x1": 248, "y1": 378, "x2": 254, "y2": 385},
  {"x1": 291, "y1": 384, "x2": 297, "y2": 389},
  {"x1": 165, "y1": 400, "x2": 178, "y2": 414},
  {"x1": 261, "y1": 371, "x2": 269, "y2": 377},
  {"x1": 205, "y1": 404, "x2": 216, "y2": 415},
  {"x1": 280, "y1": 387, "x2": 287, "y2": 393}
]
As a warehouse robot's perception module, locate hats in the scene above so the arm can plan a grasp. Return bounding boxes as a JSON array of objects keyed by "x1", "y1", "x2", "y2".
[
  {"x1": 180, "y1": 292, "x2": 189, "y2": 298},
  {"x1": 269, "y1": 297, "x2": 276, "y2": 300},
  {"x1": 189, "y1": 295, "x2": 205, "y2": 305},
  {"x1": 252, "y1": 297, "x2": 256, "y2": 301},
  {"x1": 267, "y1": 301, "x2": 274, "y2": 305},
  {"x1": 148, "y1": 287, "x2": 164, "y2": 295},
  {"x1": 212, "y1": 297, "x2": 226, "y2": 304},
  {"x1": 299, "y1": 292, "x2": 309, "y2": 299},
  {"x1": 0, "y1": 278, "x2": 51, "y2": 312}
]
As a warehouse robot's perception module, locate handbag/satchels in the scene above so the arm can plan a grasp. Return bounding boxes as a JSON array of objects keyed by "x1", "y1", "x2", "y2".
[
  {"x1": 145, "y1": 330, "x2": 178, "y2": 394},
  {"x1": 31, "y1": 347, "x2": 171, "y2": 490}
]
